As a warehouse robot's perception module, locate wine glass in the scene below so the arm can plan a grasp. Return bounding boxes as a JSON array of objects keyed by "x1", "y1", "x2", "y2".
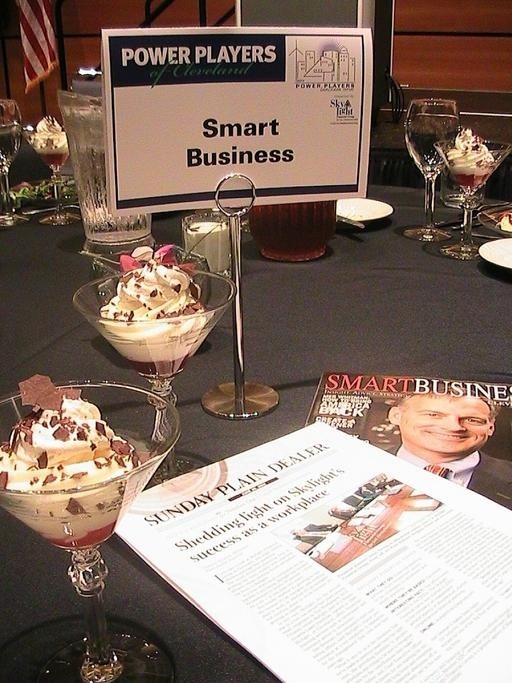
[
  {"x1": 430, "y1": 135, "x2": 511, "y2": 259},
  {"x1": 1, "y1": 381, "x2": 183, "y2": 682},
  {"x1": 398, "y1": 95, "x2": 459, "y2": 241},
  {"x1": 0, "y1": 99, "x2": 31, "y2": 226},
  {"x1": 72, "y1": 268, "x2": 233, "y2": 486},
  {"x1": 20, "y1": 126, "x2": 82, "y2": 226}
]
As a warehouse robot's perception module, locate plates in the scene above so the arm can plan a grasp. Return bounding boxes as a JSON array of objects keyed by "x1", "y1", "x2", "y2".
[
  {"x1": 334, "y1": 195, "x2": 395, "y2": 229},
  {"x1": 471, "y1": 203, "x2": 510, "y2": 235},
  {"x1": 474, "y1": 238, "x2": 512, "y2": 272}
]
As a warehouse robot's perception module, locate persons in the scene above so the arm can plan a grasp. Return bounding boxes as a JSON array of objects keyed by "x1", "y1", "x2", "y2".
[
  {"x1": 360, "y1": 486, "x2": 374, "y2": 498},
  {"x1": 290, "y1": 524, "x2": 335, "y2": 546},
  {"x1": 378, "y1": 386, "x2": 511, "y2": 512}
]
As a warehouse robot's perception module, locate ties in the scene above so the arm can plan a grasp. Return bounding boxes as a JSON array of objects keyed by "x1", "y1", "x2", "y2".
[{"x1": 425, "y1": 465, "x2": 449, "y2": 478}]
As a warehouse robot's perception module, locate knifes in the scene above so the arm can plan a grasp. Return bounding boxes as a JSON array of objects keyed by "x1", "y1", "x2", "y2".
[
  {"x1": 434, "y1": 201, "x2": 509, "y2": 227},
  {"x1": 335, "y1": 209, "x2": 366, "y2": 229}
]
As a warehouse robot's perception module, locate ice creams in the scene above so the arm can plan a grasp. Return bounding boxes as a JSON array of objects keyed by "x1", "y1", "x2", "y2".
[
  {"x1": 31, "y1": 114, "x2": 69, "y2": 166},
  {"x1": 447, "y1": 127, "x2": 496, "y2": 188},
  {"x1": 98, "y1": 259, "x2": 214, "y2": 375},
  {"x1": 0, "y1": 375, "x2": 142, "y2": 547}
]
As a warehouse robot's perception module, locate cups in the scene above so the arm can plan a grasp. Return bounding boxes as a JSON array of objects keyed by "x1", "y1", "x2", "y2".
[
  {"x1": 247, "y1": 200, "x2": 336, "y2": 261},
  {"x1": 178, "y1": 213, "x2": 232, "y2": 279},
  {"x1": 438, "y1": 159, "x2": 487, "y2": 210},
  {"x1": 53, "y1": 87, "x2": 159, "y2": 255}
]
